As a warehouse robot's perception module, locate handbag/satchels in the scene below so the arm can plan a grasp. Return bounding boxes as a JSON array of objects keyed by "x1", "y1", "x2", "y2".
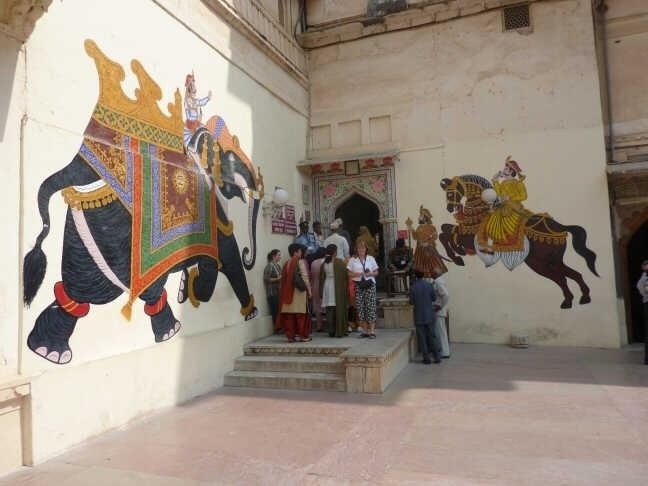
[
  {"x1": 360, "y1": 279, "x2": 373, "y2": 288},
  {"x1": 294, "y1": 273, "x2": 306, "y2": 292}
]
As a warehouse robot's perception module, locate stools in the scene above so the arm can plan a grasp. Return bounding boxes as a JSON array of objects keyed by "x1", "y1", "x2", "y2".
[{"x1": 389, "y1": 271, "x2": 408, "y2": 298}]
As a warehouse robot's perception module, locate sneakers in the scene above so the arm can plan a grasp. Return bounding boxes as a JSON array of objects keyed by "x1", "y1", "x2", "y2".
[
  {"x1": 480, "y1": 248, "x2": 493, "y2": 254},
  {"x1": 288, "y1": 338, "x2": 296, "y2": 342},
  {"x1": 317, "y1": 327, "x2": 376, "y2": 339},
  {"x1": 422, "y1": 355, "x2": 450, "y2": 364},
  {"x1": 300, "y1": 337, "x2": 312, "y2": 342}
]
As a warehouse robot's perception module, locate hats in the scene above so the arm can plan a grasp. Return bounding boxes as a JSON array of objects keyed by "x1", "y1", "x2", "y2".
[
  {"x1": 330, "y1": 218, "x2": 343, "y2": 230},
  {"x1": 506, "y1": 156, "x2": 521, "y2": 174},
  {"x1": 185, "y1": 75, "x2": 193, "y2": 86},
  {"x1": 420, "y1": 205, "x2": 431, "y2": 218},
  {"x1": 300, "y1": 221, "x2": 309, "y2": 226}
]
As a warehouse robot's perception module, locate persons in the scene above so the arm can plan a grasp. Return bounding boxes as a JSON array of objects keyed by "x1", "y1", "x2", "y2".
[
  {"x1": 310, "y1": 247, "x2": 327, "y2": 332},
  {"x1": 345, "y1": 240, "x2": 380, "y2": 339},
  {"x1": 407, "y1": 270, "x2": 441, "y2": 364},
  {"x1": 636, "y1": 259, "x2": 648, "y2": 365},
  {"x1": 182, "y1": 67, "x2": 213, "y2": 144},
  {"x1": 293, "y1": 217, "x2": 379, "y2": 272},
  {"x1": 263, "y1": 248, "x2": 284, "y2": 323},
  {"x1": 431, "y1": 266, "x2": 450, "y2": 359},
  {"x1": 343, "y1": 242, "x2": 363, "y2": 333},
  {"x1": 479, "y1": 155, "x2": 529, "y2": 254},
  {"x1": 406, "y1": 206, "x2": 446, "y2": 277},
  {"x1": 319, "y1": 244, "x2": 348, "y2": 338},
  {"x1": 277, "y1": 245, "x2": 312, "y2": 343}
]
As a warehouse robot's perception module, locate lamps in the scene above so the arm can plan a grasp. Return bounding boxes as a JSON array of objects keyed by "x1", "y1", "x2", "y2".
[
  {"x1": 263, "y1": 186, "x2": 289, "y2": 210},
  {"x1": 481, "y1": 188, "x2": 500, "y2": 208}
]
